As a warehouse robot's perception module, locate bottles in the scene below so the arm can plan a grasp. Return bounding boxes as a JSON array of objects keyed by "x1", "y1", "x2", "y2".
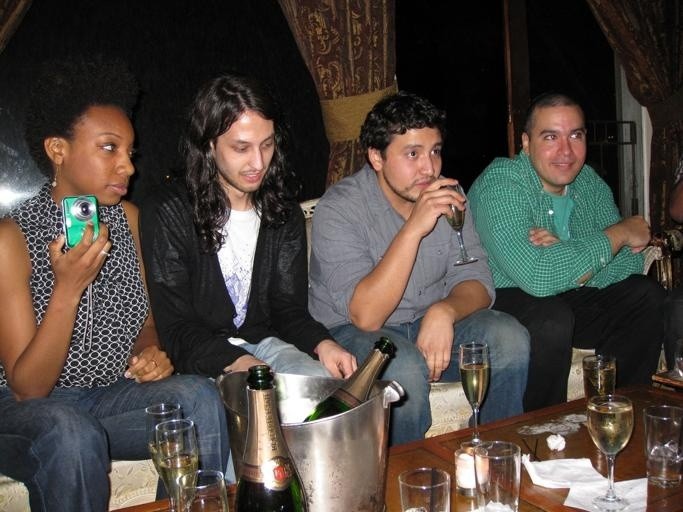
[{"x1": 235, "y1": 336, "x2": 395, "y2": 508}]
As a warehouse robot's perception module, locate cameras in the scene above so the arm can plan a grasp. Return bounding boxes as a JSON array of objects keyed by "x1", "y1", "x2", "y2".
[{"x1": 60, "y1": 195, "x2": 99, "y2": 249}]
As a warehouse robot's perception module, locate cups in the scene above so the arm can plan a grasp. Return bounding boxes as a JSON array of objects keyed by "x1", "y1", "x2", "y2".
[
  {"x1": 175, "y1": 470, "x2": 226, "y2": 510},
  {"x1": 643, "y1": 406, "x2": 683, "y2": 486},
  {"x1": 583, "y1": 355, "x2": 616, "y2": 396},
  {"x1": 400, "y1": 442, "x2": 523, "y2": 510}
]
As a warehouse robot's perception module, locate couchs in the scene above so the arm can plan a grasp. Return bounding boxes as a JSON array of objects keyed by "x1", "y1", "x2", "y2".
[{"x1": 1, "y1": 198, "x2": 665, "y2": 511}]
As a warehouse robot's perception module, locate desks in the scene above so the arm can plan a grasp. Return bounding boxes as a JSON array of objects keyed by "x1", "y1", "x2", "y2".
[{"x1": 112, "y1": 379, "x2": 682, "y2": 512}]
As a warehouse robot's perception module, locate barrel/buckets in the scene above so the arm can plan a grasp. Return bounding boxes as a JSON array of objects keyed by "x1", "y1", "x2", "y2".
[{"x1": 206, "y1": 369, "x2": 406, "y2": 512}]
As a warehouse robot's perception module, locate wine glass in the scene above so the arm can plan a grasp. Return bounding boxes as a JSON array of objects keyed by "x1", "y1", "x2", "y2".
[
  {"x1": 439, "y1": 184, "x2": 478, "y2": 265},
  {"x1": 458, "y1": 342, "x2": 493, "y2": 448},
  {"x1": 145, "y1": 401, "x2": 197, "y2": 507},
  {"x1": 588, "y1": 399, "x2": 632, "y2": 510}
]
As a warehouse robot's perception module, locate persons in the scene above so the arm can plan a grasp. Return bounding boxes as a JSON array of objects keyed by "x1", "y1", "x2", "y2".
[
  {"x1": 468, "y1": 92, "x2": 666, "y2": 412},
  {"x1": 2, "y1": 62, "x2": 232, "y2": 512},
  {"x1": 143, "y1": 73, "x2": 360, "y2": 378},
  {"x1": 665, "y1": 163, "x2": 682, "y2": 371},
  {"x1": 308, "y1": 93, "x2": 530, "y2": 443}
]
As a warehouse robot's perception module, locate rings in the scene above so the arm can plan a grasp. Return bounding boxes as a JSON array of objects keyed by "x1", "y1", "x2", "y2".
[
  {"x1": 102, "y1": 250, "x2": 110, "y2": 256},
  {"x1": 151, "y1": 360, "x2": 158, "y2": 367}
]
